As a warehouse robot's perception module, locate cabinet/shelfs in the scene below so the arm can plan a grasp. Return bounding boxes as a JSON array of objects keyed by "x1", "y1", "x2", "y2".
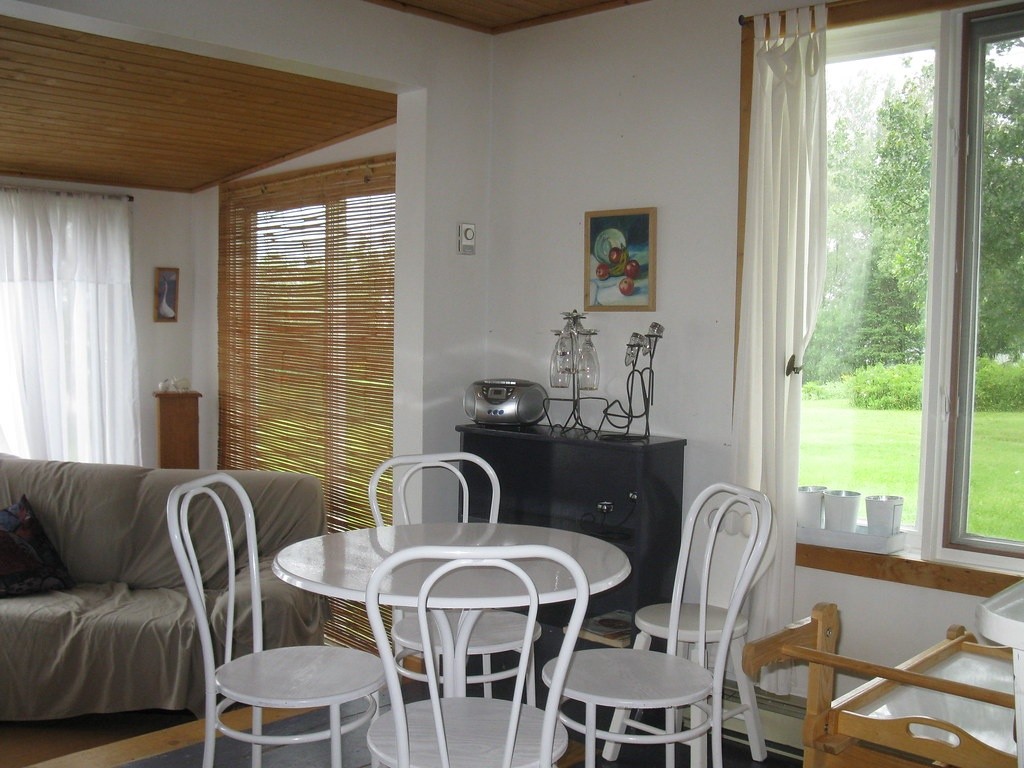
[{"x1": 455, "y1": 419, "x2": 686, "y2": 655}]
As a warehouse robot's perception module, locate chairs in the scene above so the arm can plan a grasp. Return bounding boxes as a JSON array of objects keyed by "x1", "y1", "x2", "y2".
[
  {"x1": 365, "y1": 451, "x2": 778, "y2": 768},
  {"x1": 166, "y1": 471, "x2": 386, "y2": 768}
]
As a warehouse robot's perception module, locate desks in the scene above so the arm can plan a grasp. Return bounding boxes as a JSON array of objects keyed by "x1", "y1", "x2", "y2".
[
  {"x1": 154, "y1": 391, "x2": 202, "y2": 471},
  {"x1": 269, "y1": 523, "x2": 631, "y2": 699}
]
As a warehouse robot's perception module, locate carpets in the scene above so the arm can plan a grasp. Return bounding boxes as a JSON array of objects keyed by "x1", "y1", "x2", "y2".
[{"x1": 123, "y1": 678, "x2": 804, "y2": 768}]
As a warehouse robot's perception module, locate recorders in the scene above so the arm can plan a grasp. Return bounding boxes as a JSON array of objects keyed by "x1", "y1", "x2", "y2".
[{"x1": 463, "y1": 378, "x2": 550, "y2": 427}]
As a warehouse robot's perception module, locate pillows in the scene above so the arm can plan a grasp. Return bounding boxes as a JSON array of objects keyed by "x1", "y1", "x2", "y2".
[{"x1": 0, "y1": 494, "x2": 74, "y2": 602}]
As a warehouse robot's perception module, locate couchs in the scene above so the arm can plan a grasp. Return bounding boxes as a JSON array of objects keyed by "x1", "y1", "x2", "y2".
[{"x1": 0, "y1": 450, "x2": 332, "y2": 722}]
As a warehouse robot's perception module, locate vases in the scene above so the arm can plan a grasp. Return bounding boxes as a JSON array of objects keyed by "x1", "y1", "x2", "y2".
[
  {"x1": 796, "y1": 485, "x2": 827, "y2": 530},
  {"x1": 823, "y1": 490, "x2": 862, "y2": 533},
  {"x1": 865, "y1": 495, "x2": 904, "y2": 538}
]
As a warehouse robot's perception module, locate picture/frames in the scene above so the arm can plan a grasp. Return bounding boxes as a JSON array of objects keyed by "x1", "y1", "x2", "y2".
[
  {"x1": 582, "y1": 206, "x2": 658, "y2": 314},
  {"x1": 154, "y1": 267, "x2": 180, "y2": 323}
]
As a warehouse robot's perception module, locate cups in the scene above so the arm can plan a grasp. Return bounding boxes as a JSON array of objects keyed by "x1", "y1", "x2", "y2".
[
  {"x1": 796, "y1": 485, "x2": 827, "y2": 531},
  {"x1": 823, "y1": 489, "x2": 862, "y2": 534},
  {"x1": 865, "y1": 495, "x2": 903, "y2": 538}
]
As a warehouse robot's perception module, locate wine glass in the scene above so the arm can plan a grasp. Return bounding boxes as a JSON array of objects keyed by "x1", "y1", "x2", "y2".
[
  {"x1": 576, "y1": 328, "x2": 600, "y2": 391},
  {"x1": 548, "y1": 329, "x2": 573, "y2": 388},
  {"x1": 560, "y1": 312, "x2": 588, "y2": 373}
]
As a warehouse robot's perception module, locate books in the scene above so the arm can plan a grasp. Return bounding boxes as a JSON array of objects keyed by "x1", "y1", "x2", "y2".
[{"x1": 563, "y1": 608, "x2": 632, "y2": 648}]
